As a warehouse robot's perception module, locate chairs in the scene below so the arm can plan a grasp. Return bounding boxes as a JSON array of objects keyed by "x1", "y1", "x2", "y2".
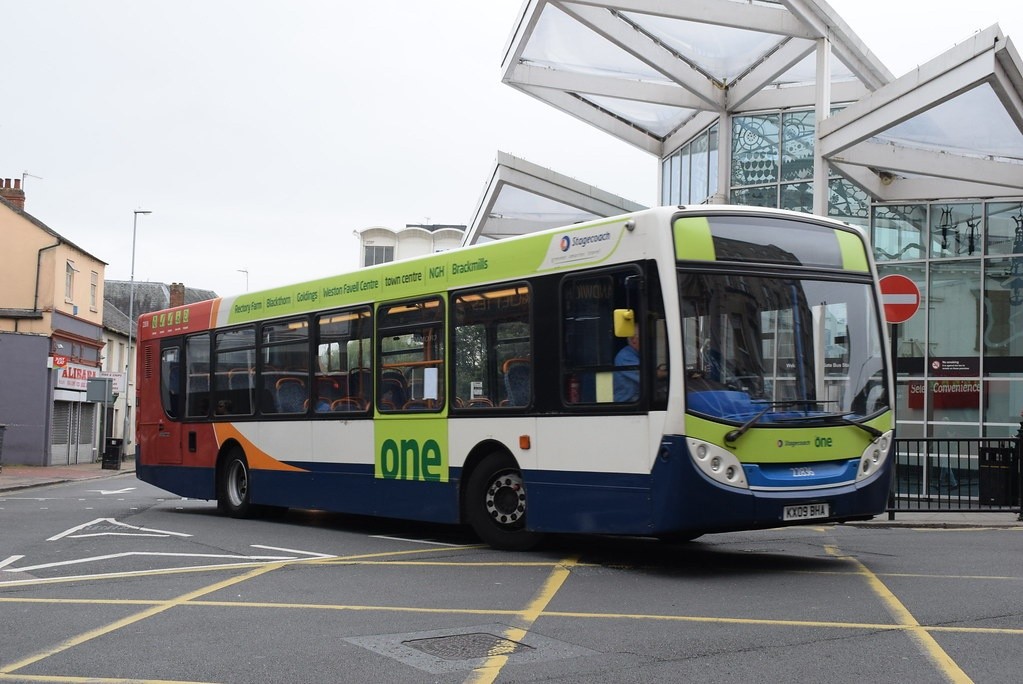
[{"x1": 166, "y1": 352, "x2": 533, "y2": 415}]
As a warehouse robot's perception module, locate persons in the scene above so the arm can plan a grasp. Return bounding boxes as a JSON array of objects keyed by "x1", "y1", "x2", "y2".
[
  {"x1": 612, "y1": 323, "x2": 668, "y2": 403},
  {"x1": 936, "y1": 468, "x2": 958, "y2": 491}
]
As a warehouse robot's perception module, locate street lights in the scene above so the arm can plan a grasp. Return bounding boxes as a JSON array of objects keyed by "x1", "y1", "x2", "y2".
[
  {"x1": 237, "y1": 269, "x2": 249, "y2": 292},
  {"x1": 122, "y1": 210, "x2": 153, "y2": 462}
]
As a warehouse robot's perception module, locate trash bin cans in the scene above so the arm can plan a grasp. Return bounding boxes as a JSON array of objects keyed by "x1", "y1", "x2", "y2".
[
  {"x1": 978, "y1": 447, "x2": 1019, "y2": 506},
  {"x1": 102, "y1": 437, "x2": 123, "y2": 470}
]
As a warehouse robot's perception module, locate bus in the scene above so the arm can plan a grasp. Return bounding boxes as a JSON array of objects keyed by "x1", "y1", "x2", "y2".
[{"x1": 135, "y1": 205, "x2": 895, "y2": 552}]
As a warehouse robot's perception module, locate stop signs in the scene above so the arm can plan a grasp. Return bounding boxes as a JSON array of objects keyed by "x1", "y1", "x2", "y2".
[{"x1": 876, "y1": 274, "x2": 920, "y2": 324}]
